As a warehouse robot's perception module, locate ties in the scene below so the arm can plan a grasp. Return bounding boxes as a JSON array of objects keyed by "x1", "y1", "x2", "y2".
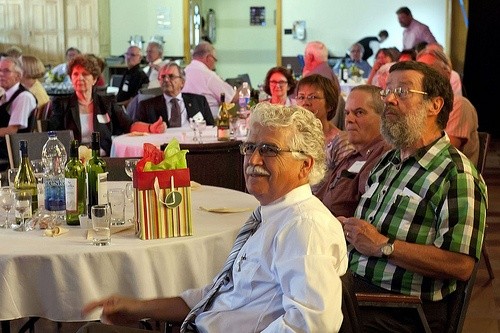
[
  {"x1": 181, "y1": 205, "x2": 262, "y2": 333},
  {"x1": 168, "y1": 98, "x2": 181, "y2": 128}
]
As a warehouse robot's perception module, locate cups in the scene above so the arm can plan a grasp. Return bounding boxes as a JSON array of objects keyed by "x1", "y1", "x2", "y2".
[
  {"x1": 106, "y1": 187, "x2": 126, "y2": 225},
  {"x1": 91, "y1": 204, "x2": 111, "y2": 245},
  {"x1": 31, "y1": 160, "x2": 42, "y2": 182},
  {"x1": 8, "y1": 168, "x2": 20, "y2": 187}
]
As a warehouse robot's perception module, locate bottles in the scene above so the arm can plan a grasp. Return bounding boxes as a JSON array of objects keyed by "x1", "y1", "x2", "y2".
[
  {"x1": 88, "y1": 132, "x2": 108, "y2": 219},
  {"x1": 13, "y1": 141, "x2": 39, "y2": 225},
  {"x1": 239, "y1": 81, "x2": 250, "y2": 105},
  {"x1": 216, "y1": 92, "x2": 230, "y2": 140},
  {"x1": 65, "y1": 140, "x2": 87, "y2": 226},
  {"x1": 42, "y1": 131, "x2": 68, "y2": 210},
  {"x1": 250, "y1": 89, "x2": 260, "y2": 109},
  {"x1": 340, "y1": 55, "x2": 361, "y2": 84}
]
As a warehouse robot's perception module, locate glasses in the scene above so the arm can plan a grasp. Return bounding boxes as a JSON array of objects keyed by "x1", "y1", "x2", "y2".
[
  {"x1": 294, "y1": 94, "x2": 323, "y2": 103},
  {"x1": 379, "y1": 86, "x2": 430, "y2": 98},
  {"x1": 239, "y1": 142, "x2": 304, "y2": 158},
  {"x1": 157, "y1": 72, "x2": 182, "y2": 81},
  {"x1": 268, "y1": 79, "x2": 289, "y2": 87}
]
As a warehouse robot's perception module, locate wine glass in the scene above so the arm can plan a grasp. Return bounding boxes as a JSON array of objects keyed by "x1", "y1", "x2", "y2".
[
  {"x1": 195, "y1": 120, "x2": 207, "y2": 143},
  {"x1": 0, "y1": 186, "x2": 17, "y2": 229},
  {"x1": 189, "y1": 116, "x2": 199, "y2": 143},
  {"x1": 126, "y1": 183, "x2": 135, "y2": 224},
  {"x1": 13, "y1": 191, "x2": 32, "y2": 232},
  {"x1": 230, "y1": 109, "x2": 252, "y2": 140},
  {"x1": 124, "y1": 158, "x2": 140, "y2": 183}
]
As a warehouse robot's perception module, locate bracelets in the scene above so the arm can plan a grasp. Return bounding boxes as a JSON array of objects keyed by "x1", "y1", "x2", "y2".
[{"x1": 148, "y1": 124, "x2": 151, "y2": 133}]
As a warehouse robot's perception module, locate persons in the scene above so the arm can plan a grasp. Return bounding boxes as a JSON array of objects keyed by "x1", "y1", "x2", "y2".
[
  {"x1": 310, "y1": 85, "x2": 392, "y2": 218},
  {"x1": 396, "y1": 7, "x2": 437, "y2": 50},
  {"x1": 337, "y1": 60, "x2": 488, "y2": 333},
  {"x1": 259, "y1": 31, "x2": 480, "y2": 168},
  {"x1": 0, "y1": 40, "x2": 243, "y2": 172},
  {"x1": 76, "y1": 102, "x2": 348, "y2": 333}
]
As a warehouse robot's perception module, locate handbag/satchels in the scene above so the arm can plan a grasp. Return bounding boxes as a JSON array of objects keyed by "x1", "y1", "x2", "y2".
[{"x1": 132, "y1": 168, "x2": 193, "y2": 241}]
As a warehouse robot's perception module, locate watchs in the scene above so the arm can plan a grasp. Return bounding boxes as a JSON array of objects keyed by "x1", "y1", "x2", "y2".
[{"x1": 381, "y1": 238, "x2": 395, "y2": 262}]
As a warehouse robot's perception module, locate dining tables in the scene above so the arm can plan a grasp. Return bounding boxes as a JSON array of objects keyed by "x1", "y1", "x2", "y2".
[
  {"x1": 0, "y1": 180, "x2": 262, "y2": 333},
  {"x1": 110, "y1": 121, "x2": 247, "y2": 158}
]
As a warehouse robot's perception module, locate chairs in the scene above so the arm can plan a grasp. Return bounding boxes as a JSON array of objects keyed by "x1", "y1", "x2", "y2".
[
  {"x1": 28, "y1": 102, "x2": 54, "y2": 133},
  {"x1": 5, "y1": 131, "x2": 75, "y2": 170},
  {"x1": 476, "y1": 131, "x2": 494, "y2": 283},
  {"x1": 108, "y1": 66, "x2": 127, "y2": 87},
  {"x1": 341, "y1": 262, "x2": 477, "y2": 333},
  {"x1": 161, "y1": 138, "x2": 244, "y2": 190}
]
